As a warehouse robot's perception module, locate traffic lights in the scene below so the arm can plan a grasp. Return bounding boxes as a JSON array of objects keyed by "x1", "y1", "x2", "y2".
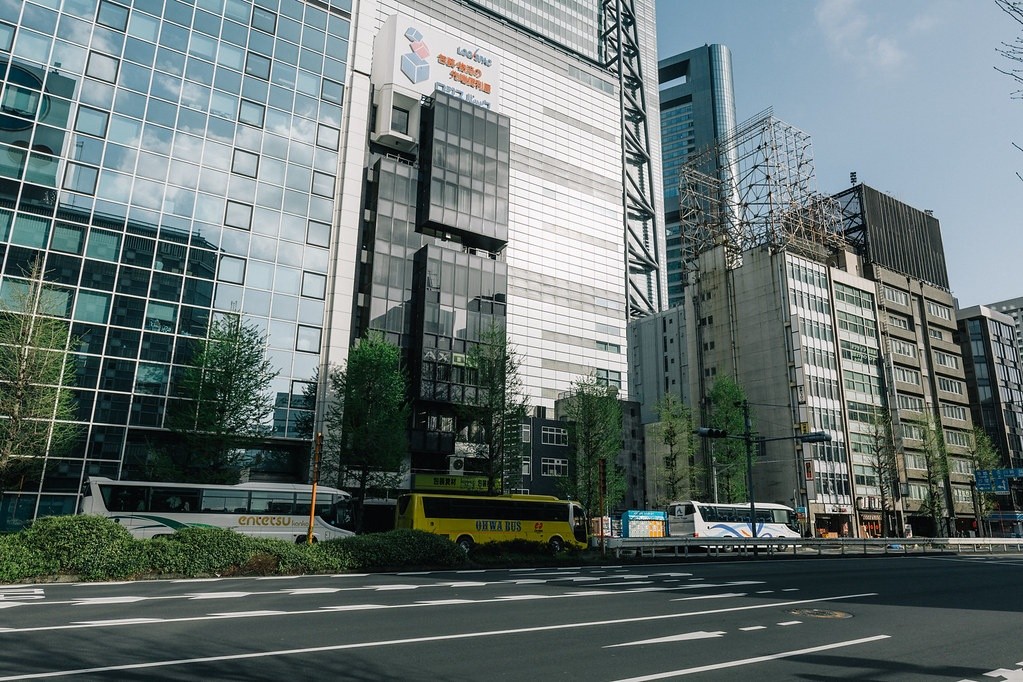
[
  {"x1": 698, "y1": 427, "x2": 727, "y2": 438},
  {"x1": 805, "y1": 462, "x2": 813, "y2": 480}
]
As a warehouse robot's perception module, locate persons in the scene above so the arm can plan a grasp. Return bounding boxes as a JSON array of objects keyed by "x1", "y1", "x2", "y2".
[{"x1": 165, "y1": 490, "x2": 182, "y2": 513}]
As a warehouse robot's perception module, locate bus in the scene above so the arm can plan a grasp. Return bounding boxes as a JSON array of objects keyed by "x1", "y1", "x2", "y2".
[
  {"x1": 396, "y1": 494, "x2": 589, "y2": 553},
  {"x1": 668, "y1": 500, "x2": 803, "y2": 552},
  {"x1": 80, "y1": 476, "x2": 356, "y2": 543}
]
